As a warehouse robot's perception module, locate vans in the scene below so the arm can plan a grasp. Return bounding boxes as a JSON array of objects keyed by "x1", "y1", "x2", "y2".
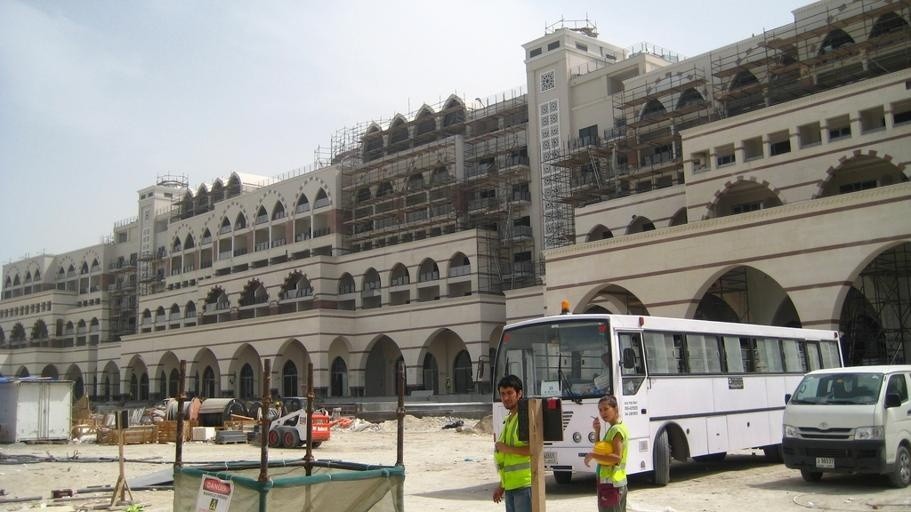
[{"x1": 781, "y1": 365, "x2": 910, "y2": 488}]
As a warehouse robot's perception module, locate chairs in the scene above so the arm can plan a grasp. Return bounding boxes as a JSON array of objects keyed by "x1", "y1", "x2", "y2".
[{"x1": 826, "y1": 379, "x2": 848, "y2": 401}]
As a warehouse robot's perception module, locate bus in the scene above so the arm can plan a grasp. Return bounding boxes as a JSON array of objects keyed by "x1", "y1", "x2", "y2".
[{"x1": 492, "y1": 300, "x2": 844, "y2": 486}]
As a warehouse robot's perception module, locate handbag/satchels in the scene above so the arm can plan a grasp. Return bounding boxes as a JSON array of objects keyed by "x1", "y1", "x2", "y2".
[{"x1": 596, "y1": 483, "x2": 620, "y2": 507}]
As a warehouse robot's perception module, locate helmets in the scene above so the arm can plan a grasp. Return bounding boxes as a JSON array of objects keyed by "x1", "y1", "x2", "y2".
[{"x1": 592, "y1": 441, "x2": 618, "y2": 466}]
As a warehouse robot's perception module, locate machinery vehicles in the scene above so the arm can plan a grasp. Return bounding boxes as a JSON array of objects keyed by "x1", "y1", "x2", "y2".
[{"x1": 255, "y1": 396, "x2": 330, "y2": 448}]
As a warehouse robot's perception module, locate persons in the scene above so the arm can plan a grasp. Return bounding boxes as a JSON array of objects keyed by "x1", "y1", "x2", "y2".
[
  {"x1": 601, "y1": 353, "x2": 610, "y2": 393},
  {"x1": 492, "y1": 375, "x2": 533, "y2": 511},
  {"x1": 585, "y1": 396, "x2": 629, "y2": 512}
]
